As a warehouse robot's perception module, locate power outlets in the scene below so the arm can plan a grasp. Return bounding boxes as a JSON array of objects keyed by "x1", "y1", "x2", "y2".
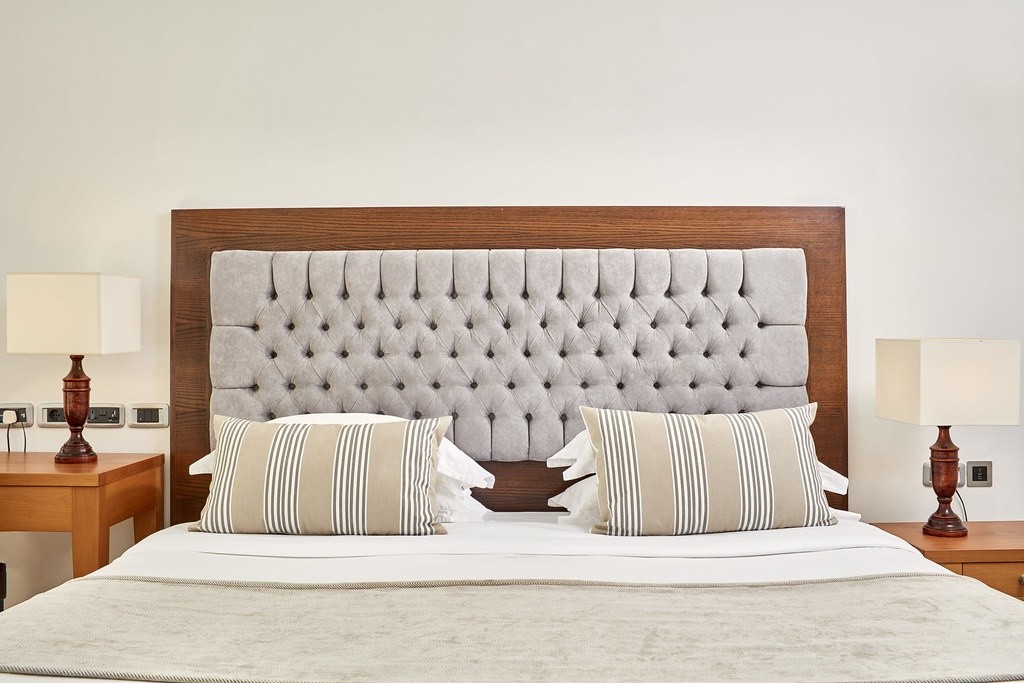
[
  {"x1": 0, "y1": 404, "x2": 34, "y2": 427},
  {"x1": 38, "y1": 403, "x2": 125, "y2": 428},
  {"x1": 923, "y1": 462, "x2": 965, "y2": 487}
]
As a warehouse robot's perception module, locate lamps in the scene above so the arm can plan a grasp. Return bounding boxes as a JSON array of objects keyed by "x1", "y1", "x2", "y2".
[
  {"x1": 4, "y1": 272, "x2": 140, "y2": 463},
  {"x1": 876, "y1": 339, "x2": 1018, "y2": 537}
]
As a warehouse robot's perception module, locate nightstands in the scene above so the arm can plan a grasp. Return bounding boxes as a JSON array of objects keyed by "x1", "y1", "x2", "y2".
[{"x1": 871, "y1": 520, "x2": 1024, "y2": 602}]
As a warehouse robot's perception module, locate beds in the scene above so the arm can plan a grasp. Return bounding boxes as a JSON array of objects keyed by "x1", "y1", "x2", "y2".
[{"x1": 0, "y1": 207, "x2": 1024, "y2": 683}]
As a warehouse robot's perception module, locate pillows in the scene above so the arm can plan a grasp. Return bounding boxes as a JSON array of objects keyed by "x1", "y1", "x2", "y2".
[
  {"x1": 188, "y1": 412, "x2": 495, "y2": 536},
  {"x1": 548, "y1": 402, "x2": 849, "y2": 536}
]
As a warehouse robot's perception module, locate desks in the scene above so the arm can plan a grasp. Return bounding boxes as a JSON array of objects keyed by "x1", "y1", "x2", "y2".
[{"x1": 0, "y1": 450, "x2": 165, "y2": 577}]
